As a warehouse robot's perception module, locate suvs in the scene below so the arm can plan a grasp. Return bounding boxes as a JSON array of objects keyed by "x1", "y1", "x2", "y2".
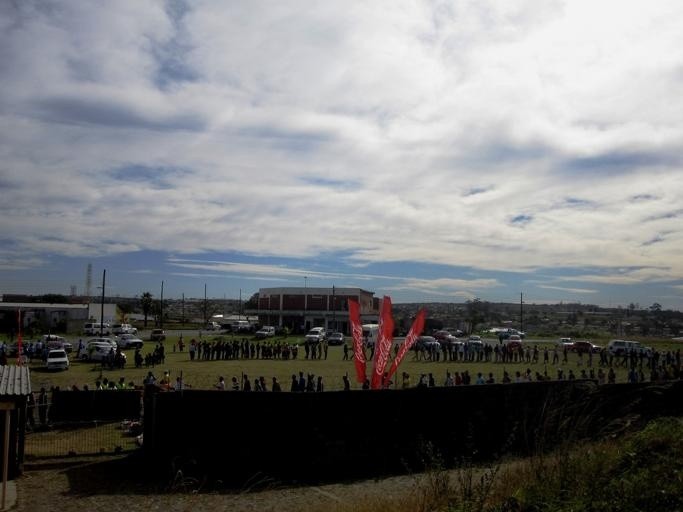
[
  {"x1": 304, "y1": 327, "x2": 325, "y2": 342},
  {"x1": 557, "y1": 336, "x2": 652, "y2": 356}
]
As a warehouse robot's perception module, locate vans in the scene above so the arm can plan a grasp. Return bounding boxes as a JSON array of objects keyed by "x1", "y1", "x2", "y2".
[{"x1": 231, "y1": 320, "x2": 249, "y2": 330}]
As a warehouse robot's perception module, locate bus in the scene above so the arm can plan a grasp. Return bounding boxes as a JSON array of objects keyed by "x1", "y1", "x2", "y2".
[{"x1": 359, "y1": 323, "x2": 378, "y2": 345}]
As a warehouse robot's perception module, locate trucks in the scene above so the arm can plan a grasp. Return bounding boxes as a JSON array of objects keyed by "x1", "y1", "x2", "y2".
[{"x1": 254, "y1": 325, "x2": 274, "y2": 338}]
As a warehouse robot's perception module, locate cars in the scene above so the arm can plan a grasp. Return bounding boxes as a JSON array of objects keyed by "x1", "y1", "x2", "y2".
[
  {"x1": 0, "y1": 321, "x2": 166, "y2": 370},
  {"x1": 327, "y1": 332, "x2": 343, "y2": 344},
  {"x1": 205, "y1": 321, "x2": 221, "y2": 331},
  {"x1": 414, "y1": 327, "x2": 525, "y2": 351}
]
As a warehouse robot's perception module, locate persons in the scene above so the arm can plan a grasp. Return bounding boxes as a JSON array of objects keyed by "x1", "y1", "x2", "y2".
[
  {"x1": 536, "y1": 344, "x2": 682, "y2": 384},
  {"x1": 75, "y1": 338, "x2": 85, "y2": 358},
  {"x1": 25, "y1": 385, "x2": 61, "y2": 424},
  {"x1": 351, "y1": 335, "x2": 402, "y2": 361},
  {"x1": 2, "y1": 329, "x2": 72, "y2": 366},
  {"x1": 68, "y1": 371, "x2": 185, "y2": 392},
  {"x1": 413, "y1": 341, "x2": 540, "y2": 364},
  {"x1": 214, "y1": 371, "x2": 324, "y2": 392},
  {"x1": 99, "y1": 345, "x2": 127, "y2": 369},
  {"x1": 343, "y1": 368, "x2": 533, "y2": 390},
  {"x1": 305, "y1": 340, "x2": 328, "y2": 360},
  {"x1": 177, "y1": 337, "x2": 184, "y2": 352},
  {"x1": 343, "y1": 344, "x2": 348, "y2": 360},
  {"x1": 189, "y1": 338, "x2": 299, "y2": 362},
  {"x1": 135, "y1": 343, "x2": 165, "y2": 369}
]
